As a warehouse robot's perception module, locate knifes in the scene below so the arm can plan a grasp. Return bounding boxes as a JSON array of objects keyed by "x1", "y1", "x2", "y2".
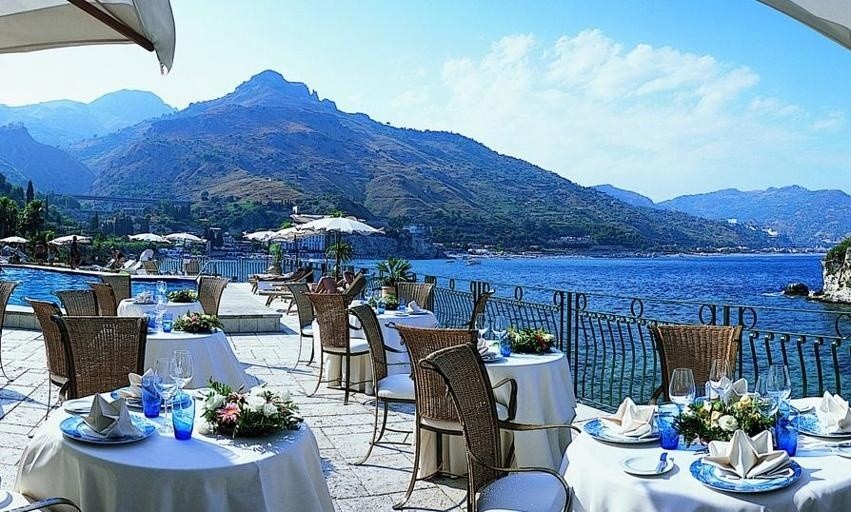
[{"x1": 656, "y1": 452, "x2": 669, "y2": 475}]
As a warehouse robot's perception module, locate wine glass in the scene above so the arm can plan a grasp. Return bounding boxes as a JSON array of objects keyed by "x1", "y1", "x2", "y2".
[
  {"x1": 170, "y1": 349, "x2": 194, "y2": 395},
  {"x1": 668, "y1": 368, "x2": 696, "y2": 416},
  {"x1": 709, "y1": 357, "x2": 734, "y2": 410},
  {"x1": 475, "y1": 313, "x2": 490, "y2": 338},
  {"x1": 491, "y1": 315, "x2": 506, "y2": 355}
]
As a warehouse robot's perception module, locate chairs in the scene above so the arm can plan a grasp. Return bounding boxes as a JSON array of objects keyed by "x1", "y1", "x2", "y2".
[
  {"x1": 394, "y1": 281, "x2": 435, "y2": 314},
  {"x1": 347, "y1": 300, "x2": 417, "y2": 468},
  {"x1": 198, "y1": 275, "x2": 231, "y2": 323},
  {"x1": 85, "y1": 280, "x2": 118, "y2": 316},
  {"x1": 0, "y1": 478, "x2": 83, "y2": 512},
  {"x1": 1, "y1": 279, "x2": 21, "y2": 382},
  {"x1": 49, "y1": 287, "x2": 101, "y2": 316},
  {"x1": 299, "y1": 289, "x2": 371, "y2": 405},
  {"x1": 246, "y1": 270, "x2": 314, "y2": 308},
  {"x1": 382, "y1": 316, "x2": 518, "y2": 512},
  {"x1": 180, "y1": 260, "x2": 200, "y2": 275},
  {"x1": 48, "y1": 312, "x2": 150, "y2": 404},
  {"x1": 418, "y1": 340, "x2": 582, "y2": 512},
  {"x1": 97, "y1": 272, "x2": 133, "y2": 311},
  {"x1": 438, "y1": 287, "x2": 496, "y2": 330},
  {"x1": 21, "y1": 296, "x2": 70, "y2": 421},
  {"x1": 643, "y1": 325, "x2": 746, "y2": 410},
  {"x1": 283, "y1": 271, "x2": 370, "y2": 374},
  {"x1": 141, "y1": 261, "x2": 164, "y2": 275}
]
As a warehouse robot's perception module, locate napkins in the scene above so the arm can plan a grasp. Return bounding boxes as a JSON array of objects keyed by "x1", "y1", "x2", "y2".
[
  {"x1": 134, "y1": 291, "x2": 155, "y2": 303},
  {"x1": 79, "y1": 390, "x2": 146, "y2": 439}
]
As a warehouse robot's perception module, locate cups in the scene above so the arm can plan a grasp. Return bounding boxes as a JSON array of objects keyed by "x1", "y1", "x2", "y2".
[
  {"x1": 141, "y1": 375, "x2": 162, "y2": 417},
  {"x1": 776, "y1": 411, "x2": 798, "y2": 457},
  {"x1": 753, "y1": 364, "x2": 792, "y2": 420},
  {"x1": 376, "y1": 301, "x2": 386, "y2": 314},
  {"x1": 156, "y1": 281, "x2": 167, "y2": 294},
  {"x1": 162, "y1": 312, "x2": 173, "y2": 333},
  {"x1": 169, "y1": 395, "x2": 195, "y2": 440},
  {"x1": 657, "y1": 402, "x2": 681, "y2": 449},
  {"x1": 397, "y1": 298, "x2": 406, "y2": 312}
]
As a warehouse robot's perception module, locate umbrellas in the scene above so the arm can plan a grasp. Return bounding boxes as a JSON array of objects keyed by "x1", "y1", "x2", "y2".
[
  {"x1": 46, "y1": 235, "x2": 92, "y2": 246},
  {"x1": 1, "y1": 0, "x2": 175, "y2": 75},
  {"x1": 0, "y1": 236, "x2": 30, "y2": 243},
  {"x1": 244, "y1": 215, "x2": 386, "y2": 282},
  {"x1": 127, "y1": 233, "x2": 171, "y2": 250},
  {"x1": 163, "y1": 232, "x2": 203, "y2": 270}
]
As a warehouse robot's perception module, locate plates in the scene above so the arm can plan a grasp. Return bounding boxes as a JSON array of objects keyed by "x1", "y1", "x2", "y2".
[
  {"x1": 395, "y1": 311, "x2": 428, "y2": 316},
  {"x1": 830, "y1": 440, "x2": 851, "y2": 459},
  {"x1": 689, "y1": 455, "x2": 803, "y2": 494},
  {"x1": 110, "y1": 386, "x2": 189, "y2": 408},
  {"x1": 64, "y1": 399, "x2": 98, "y2": 413},
  {"x1": 59, "y1": 412, "x2": 156, "y2": 444},
  {"x1": 583, "y1": 418, "x2": 661, "y2": 444},
  {"x1": 621, "y1": 455, "x2": 674, "y2": 476},
  {"x1": 788, "y1": 413, "x2": 851, "y2": 437}
]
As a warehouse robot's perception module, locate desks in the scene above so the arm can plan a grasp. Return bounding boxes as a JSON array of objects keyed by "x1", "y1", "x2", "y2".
[
  {"x1": 141, "y1": 325, "x2": 252, "y2": 396},
  {"x1": 16, "y1": 384, "x2": 336, "y2": 512},
  {"x1": 566, "y1": 397, "x2": 851, "y2": 512},
  {"x1": 116, "y1": 295, "x2": 207, "y2": 319},
  {"x1": 312, "y1": 306, "x2": 442, "y2": 395}
]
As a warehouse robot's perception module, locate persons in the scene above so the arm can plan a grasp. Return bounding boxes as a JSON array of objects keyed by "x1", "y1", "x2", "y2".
[
  {"x1": 70, "y1": 236, "x2": 79, "y2": 269},
  {"x1": 109, "y1": 247, "x2": 125, "y2": 269},
  {"x1": 313, "y1": 270, "x2": 356, "y2": 294},
  {"x1": 140, "y1": 246, "x2": 156, "y2": 263},
  {"x1": 34, "y1": 241, "x2": 56, "y2": 265},
  {"x1": 3, "y1": 242, "x2": 9, "y2": 256}
]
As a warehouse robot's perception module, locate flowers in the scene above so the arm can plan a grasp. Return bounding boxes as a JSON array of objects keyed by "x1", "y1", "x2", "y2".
[
  {"x1": 181, "y1": 309, "x2": 225, "y2": 335},
  {"x1": 197, "y1": 375, "x2": 305, "y2": 442},
  {"x1": 164, "y1": 287, "x2": 198, "y2": 303}
]
